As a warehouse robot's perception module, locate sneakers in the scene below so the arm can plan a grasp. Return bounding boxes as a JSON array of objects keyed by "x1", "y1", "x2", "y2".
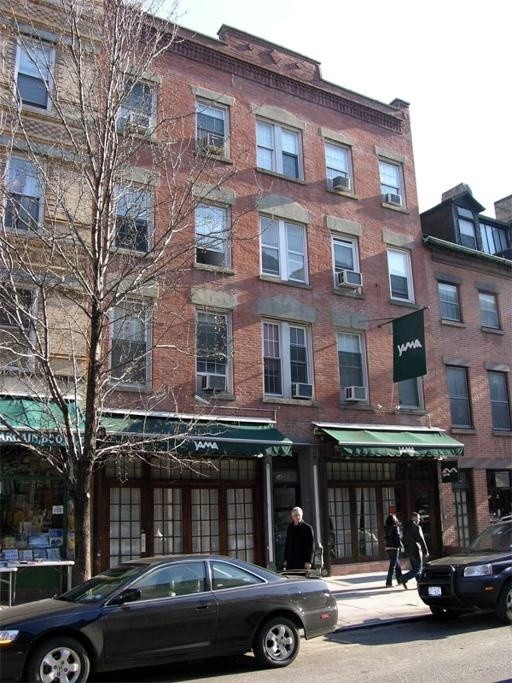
[{"x1": 385, "y1": 577, "x2": 408, "y2": 590}]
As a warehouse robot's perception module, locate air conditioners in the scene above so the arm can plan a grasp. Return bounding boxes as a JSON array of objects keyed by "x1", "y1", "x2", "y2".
[
  {"x1": 345, "y1": 386, "x2": 368, "y2": 402},
  {"x1": 292, "y1": 383, "x2": 314, "y2": 399},
  {"x1": 337, "y1": 270, "x2": 363, "y2": 289},
  {"x1": 333, "y1": 176, "x2": 350, "y2": 191},
  {"x1": 201, "y1": 132, "x2": 225, "y2": 154},
  {"x1": 201, "y1": 374, "x2": 227, "y2": 391},
  {"x1": 385, "y1": 192, "x2": 401, "y2": 207},
  {"x1": 127, "y1": 112, "x2": 150, "y2": 135}
]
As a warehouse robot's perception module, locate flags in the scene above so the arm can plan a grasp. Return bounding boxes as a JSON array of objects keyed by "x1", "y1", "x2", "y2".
[{"x1": 389, "y1": 305, "x2": 432, "y2": 383}]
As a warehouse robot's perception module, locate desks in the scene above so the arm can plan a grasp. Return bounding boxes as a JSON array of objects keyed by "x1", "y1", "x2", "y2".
[{"x1": 0, "y1": 559, "x2": 75, "y2": 606}]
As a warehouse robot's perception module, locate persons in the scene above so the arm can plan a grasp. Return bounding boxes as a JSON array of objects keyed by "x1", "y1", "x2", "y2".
[
  {"x1": 383, "y1": 512, "x2": 404, "y2": 588},
  {"x1": 398, "y1": 510, "x2": 430, "y2": 589},
  {"x1": 282, "y1": 504, "x2": 316, "y2": 578}
]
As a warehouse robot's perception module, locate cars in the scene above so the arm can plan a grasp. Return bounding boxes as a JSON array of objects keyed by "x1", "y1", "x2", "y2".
[
  {"x1": 417, "y1": 512, "x2": 512, "y2": 625},
  {"x1": 0, "y1": 552, "x2": 338, "y2": 682}
]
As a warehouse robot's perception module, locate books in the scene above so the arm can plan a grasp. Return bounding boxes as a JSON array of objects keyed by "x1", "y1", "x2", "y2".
[{"x1": 0, "y1": 521, "x2": 64, "y2": 568}]
